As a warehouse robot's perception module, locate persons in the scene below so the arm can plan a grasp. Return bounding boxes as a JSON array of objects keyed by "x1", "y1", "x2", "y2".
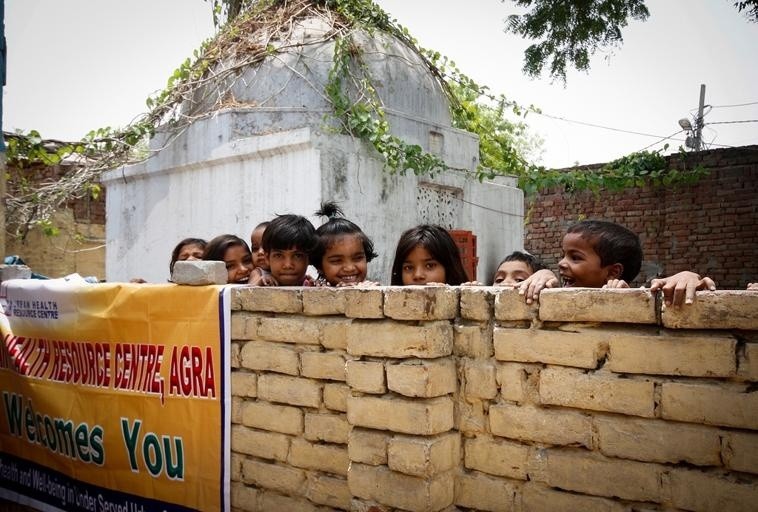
[
  {"x1": 746, "y1": 282, "x2": 758, "y2": 291},
  {"x1": 459, "y1": 250, "x2": 538, "y2": 287},
  {"x1": 243, "y1": 213, "x2": 318, "y2": 286},
  {"x1": 249, "y1": 222, "x2": 272, "y2": 273},
  {"x1": 512, "y1": 220, "x2": 643, "y2": 305},
  {"x1": 355, "y1": 224, "x2": 470, "y2": 293},
  {"x1": 649, "y1": 270, "x2": 716, "y2": 310},
  {"x1": 129, "y1": 236, "x2": 207, "y2": 284},
  {"x1": 303, "y1": 199, "x2": 377, "y2": 288},
  {"x1": 201, "y1": 234, "x2": 256, "y2": 285}
]
